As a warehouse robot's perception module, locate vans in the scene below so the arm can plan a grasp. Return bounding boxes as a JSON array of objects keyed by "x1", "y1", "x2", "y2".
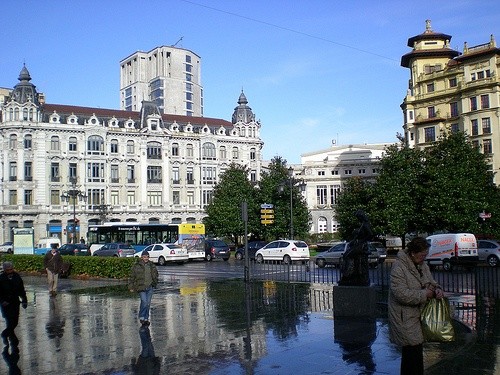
[
  {"x1": 424, "y1": 233, "x2": 478, "y2": 272},
  {"x1": 33, "y1": 239, "x2": 61, "y2": 255}
]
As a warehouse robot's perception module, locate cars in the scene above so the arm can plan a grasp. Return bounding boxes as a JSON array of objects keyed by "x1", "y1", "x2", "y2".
[
  {"x1": 93, "y1": 243, "x2": 136, "y2": 258},
  {"x1": 0, "y1": 241, "x2": 14, "y2": 253},
  {"x1": 255, "y1": 240, "x2": 310, "y2": 265},
  {"x1": 205, "y1": 240, "x2": 230, "y2": 262},
  {"x1": 134, "y1": 243, "x2": 189, "y2": 266},
  {"x1": 235, "y1": 241, "x2": 268, "y2": 260},
  {"x1": 476, "y1": 240, "x2": 500, "y2": 267},
  {"x1": 56, "y1": 244, "x2": 91, "y2": 257}
]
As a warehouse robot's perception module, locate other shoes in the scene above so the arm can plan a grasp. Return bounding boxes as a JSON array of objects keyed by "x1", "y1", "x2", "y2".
[
  {"x1": 1, "y1": 333, "x2": 9, "y2": 346},
  {"x1": 139, "y1": 317, "x2": 150, "y2": 328},
  {"x1": 54, "y1": 291, "x2": 57, "y2": 295}
]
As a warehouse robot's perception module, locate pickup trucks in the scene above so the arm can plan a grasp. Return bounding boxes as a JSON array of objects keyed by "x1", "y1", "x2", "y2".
[{"x1": 313, "y1": 242, "x2": 386, "y2": 268}]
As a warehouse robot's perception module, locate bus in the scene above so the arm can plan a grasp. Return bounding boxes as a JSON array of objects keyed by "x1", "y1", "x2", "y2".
[{"x1": 87, "y1": 224, "x2": 205, "y2": 260}]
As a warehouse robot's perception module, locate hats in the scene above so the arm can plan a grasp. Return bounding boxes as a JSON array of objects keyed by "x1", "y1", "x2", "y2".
[{"x1": 141, "y1": 251, "x2": 149, "y2": 256}]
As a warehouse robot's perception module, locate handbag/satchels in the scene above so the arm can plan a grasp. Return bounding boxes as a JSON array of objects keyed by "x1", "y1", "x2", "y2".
[{"x1": 420, "y1": 299, "x2": 455, "y2": 342}]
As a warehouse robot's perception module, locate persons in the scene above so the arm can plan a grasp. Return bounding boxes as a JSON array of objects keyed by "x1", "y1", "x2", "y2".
[
  {"x1": 128, "y1": 250, "x2": 159, "y2": 325},
  {"x1": 104, "y1": 234, "x2": 177, "y2": 245},
  {"x1": 43, "y1": 246, "x2": 63, "y2": 295},
  {"x1": 0, "y1": 261, "x2": 28, "y2": 354},
  {"x1": 388, "y1": 236, "x2": 444, "y2": 375},
  {"x1": 80, "y1": 236, "x2": 85, "y2": 245}
]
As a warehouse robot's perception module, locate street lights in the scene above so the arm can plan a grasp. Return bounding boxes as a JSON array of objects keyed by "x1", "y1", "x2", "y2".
[
  {"x1": 288, "y1": 166, "x2": 294, "y2": 240},
  {"x1": 60, "y1": 181, "x2": 87, "y2": 244}
]
